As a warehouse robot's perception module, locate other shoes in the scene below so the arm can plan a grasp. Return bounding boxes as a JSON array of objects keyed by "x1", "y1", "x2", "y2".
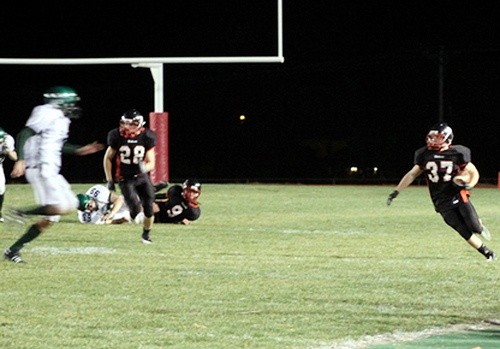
[
  {"x1": 3, "y1": 248, "x2": 25, "y2": 264},
  {"x1": 5, "y1": 208, "x2": 26, "y2": 223},
  {"x1": 140, "y1": 233, "x2": 153, "y2": 245},
  {"x1": 482, "y1": 249, "x2": 496, "y2": 261},
  {"x1": 481, "y1": 222, "x2": 490, "y2": 241}
]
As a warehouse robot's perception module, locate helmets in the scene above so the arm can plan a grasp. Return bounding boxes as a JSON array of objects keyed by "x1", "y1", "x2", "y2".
[
  {"x1": 428, "y1": 122, "x2": 454, "y2": 146},
  {"x1": 181, "y1": 178, "x2": 202, "y2": 196},
  {"x1": 42, "y1": 87, "x2": 80, "y2": 107},
  {"x1": 76, "y1": 193, "x2": 90, "y2": 212},
  {"x1": 121, "y1": 109, "x2": 144, "y2": 131}
]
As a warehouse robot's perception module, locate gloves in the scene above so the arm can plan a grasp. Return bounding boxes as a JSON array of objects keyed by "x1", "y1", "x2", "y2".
[
  {"x1": 386, "y1": 190, "x2": 399, "y2": 206},
  {"x1": 107, "y1": 181, "x2": 116, "y2": 192}
]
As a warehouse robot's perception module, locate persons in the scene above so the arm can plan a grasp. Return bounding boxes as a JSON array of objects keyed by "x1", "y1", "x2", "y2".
[
  {"x1": 0, "y1": 129, "x2": 17, "y2": 222},
  {"x1": 103, "y1": 112, "x2": 158, "y2": 243},
  {"x1": 386, "y1": 123, "x2": 495, "y2": 261},
  {"x1": 153, "y1": 178, "x2": 202, "y2": 225},
  {"x1": 76, "y1": 183, "x2": 159, "y2": 225},
  {"x1": 4, "y1": 86, "x2": 103, "y2": 262}
]
